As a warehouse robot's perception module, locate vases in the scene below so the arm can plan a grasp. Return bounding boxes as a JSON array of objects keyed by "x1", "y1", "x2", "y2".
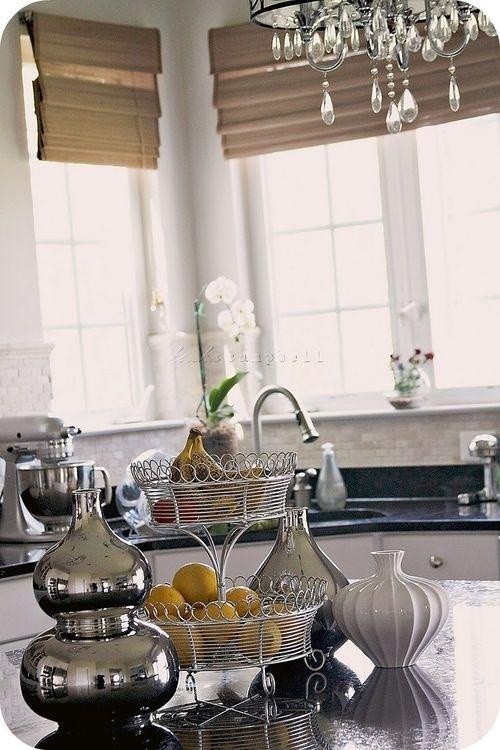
[
  {"x1": 190, "y1": 424, "x2": 240, "y2": 474},
  {"x1": 330, "y1": 549, "x2": 451, "y2": 670},
  {"x1": 389, "y1": 398, "x2": 424, "y2": 410}
]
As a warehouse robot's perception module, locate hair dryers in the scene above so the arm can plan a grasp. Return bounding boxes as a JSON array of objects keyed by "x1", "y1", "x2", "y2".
[{"x1": 316, "y1": 442, "x2": 349, "y2": 512}]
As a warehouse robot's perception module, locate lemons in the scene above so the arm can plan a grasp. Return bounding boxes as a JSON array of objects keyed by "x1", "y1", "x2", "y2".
[{"x1": 175, "y1": 708, "x2": 289, "y2": 750}]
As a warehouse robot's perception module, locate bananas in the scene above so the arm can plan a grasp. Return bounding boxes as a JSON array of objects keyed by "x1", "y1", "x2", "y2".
[{"x1": 171, "y1": 428, "x2": 265, "y2": 492}]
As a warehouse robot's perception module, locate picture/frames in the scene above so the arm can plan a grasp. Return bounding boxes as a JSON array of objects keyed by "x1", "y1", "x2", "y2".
[{"x1": 340, "y1": 666, "x2": 456, "y2": 750}]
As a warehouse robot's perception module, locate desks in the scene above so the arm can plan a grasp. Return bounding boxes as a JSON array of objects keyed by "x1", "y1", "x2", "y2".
[{"x1": 0, "y1": 579, "x2": 500, "y2": 750}]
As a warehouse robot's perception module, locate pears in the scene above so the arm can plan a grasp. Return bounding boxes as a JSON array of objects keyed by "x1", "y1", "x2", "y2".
[{"x1": 144, "y1": 563, "x2": 306, "y2": 662}]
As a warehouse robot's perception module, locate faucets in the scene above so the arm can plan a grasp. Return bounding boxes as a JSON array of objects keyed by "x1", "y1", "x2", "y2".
[{"x1": 252, "y1": 384, "x2": 317, "y2": 467}]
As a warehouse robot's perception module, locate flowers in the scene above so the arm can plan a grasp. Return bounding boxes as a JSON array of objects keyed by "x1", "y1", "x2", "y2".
[
  {"x1": 389, "y1": 347, "x2": 435, "y2": 397},
  {"x1": 193, "y1": 274, "x2": 256, "y2": 430}
]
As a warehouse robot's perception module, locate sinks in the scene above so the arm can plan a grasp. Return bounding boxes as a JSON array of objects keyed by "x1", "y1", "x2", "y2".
[{"x1": 305, "y1": 506, "x2": 387, "y2": 524}]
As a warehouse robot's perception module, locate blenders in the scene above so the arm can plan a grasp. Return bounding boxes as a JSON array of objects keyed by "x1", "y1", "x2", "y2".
[{"x1": 0, "y1": 412, "x2": 114, "y2": 544}]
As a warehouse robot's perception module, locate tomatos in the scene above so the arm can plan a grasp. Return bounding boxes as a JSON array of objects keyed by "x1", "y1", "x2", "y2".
[{"x1": 153, "y1": 497, "x2": 197, "y2": 523}]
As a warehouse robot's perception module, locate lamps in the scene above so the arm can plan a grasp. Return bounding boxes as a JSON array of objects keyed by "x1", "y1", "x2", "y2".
[{"x1": 246, "y1": 1, "x2": 500, "y2": 136}]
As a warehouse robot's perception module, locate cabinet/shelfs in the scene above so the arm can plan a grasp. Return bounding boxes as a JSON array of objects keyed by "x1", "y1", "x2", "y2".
[
  {"x1": 129, "y1": 449, "x2": 327, "y2": 696},
  {"x1": 153, "y1": 673, "x2": 332, "y2": 750}
]
